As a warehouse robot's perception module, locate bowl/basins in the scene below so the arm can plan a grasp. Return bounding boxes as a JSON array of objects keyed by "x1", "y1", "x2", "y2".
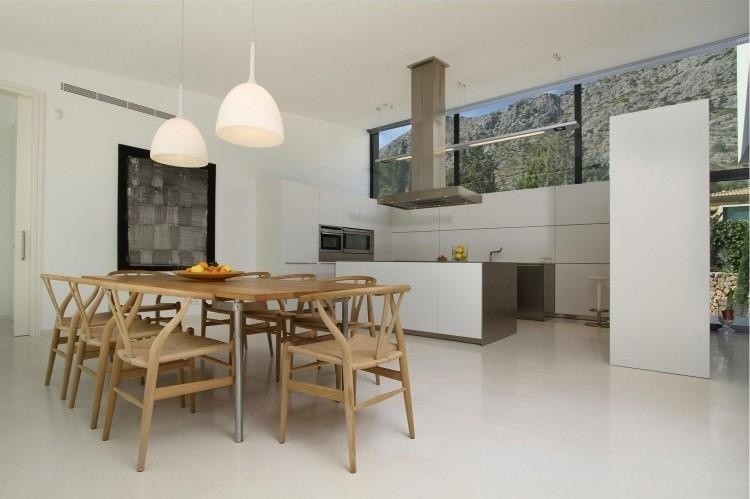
[{"x1": 451, "y1": 245, "x2": 470, "y2": 262}]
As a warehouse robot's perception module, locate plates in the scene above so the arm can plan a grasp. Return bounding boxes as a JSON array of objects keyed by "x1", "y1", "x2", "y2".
[{"x1": 171, "y1": 271, "x2": 245, "y2": 282}]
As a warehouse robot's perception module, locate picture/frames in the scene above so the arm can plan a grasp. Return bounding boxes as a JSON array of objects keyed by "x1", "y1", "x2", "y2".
[{"x1": 117, "y1": 143, "x2": 217, "y2": 272}]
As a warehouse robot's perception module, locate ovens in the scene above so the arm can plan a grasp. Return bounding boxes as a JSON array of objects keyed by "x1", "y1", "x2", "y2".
[{"x1": 319, "y1": 224, "x2": 374, "y2": 262}]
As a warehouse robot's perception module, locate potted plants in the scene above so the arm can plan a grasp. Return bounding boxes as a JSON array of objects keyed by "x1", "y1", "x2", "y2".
[{"x1": 716, "y1": 286, "x2": 735, "y2": 336}]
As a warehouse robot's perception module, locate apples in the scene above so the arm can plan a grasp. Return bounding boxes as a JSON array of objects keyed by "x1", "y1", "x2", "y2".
[{"x1": 454, "y1": 243, "x2": 467, "y2": 260}]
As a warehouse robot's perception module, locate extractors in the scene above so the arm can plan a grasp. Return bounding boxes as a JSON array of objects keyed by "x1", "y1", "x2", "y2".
[{"x1": 377, "y1": 56, "x2": 483, "y2": 212}]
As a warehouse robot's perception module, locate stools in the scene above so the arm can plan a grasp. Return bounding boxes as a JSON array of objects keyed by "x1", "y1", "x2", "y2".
[{"x1": 584, "y1": 274, "x2": 610, "y2": 328}]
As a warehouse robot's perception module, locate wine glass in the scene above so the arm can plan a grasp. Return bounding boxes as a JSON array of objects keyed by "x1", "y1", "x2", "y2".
[{"x1": 715, "y1": 298, "x2": 737, "y2": 334}]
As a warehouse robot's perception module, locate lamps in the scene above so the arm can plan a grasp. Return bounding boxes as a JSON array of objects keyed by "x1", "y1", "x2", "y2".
[
  {"x1": 149, "y1": 0, "x2": 209, "y2": 169},
  {"x1": 374, "y1": 56, "x2": 581, "y2": 166},
  {"x1": 213, "y1": 0, "x2": 285, "y2": 150}
]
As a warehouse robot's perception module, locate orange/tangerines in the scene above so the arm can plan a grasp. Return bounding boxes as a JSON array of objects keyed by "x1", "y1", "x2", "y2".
[{"x1": 186, "y1": 262, "x2": 232, "y2": 274}]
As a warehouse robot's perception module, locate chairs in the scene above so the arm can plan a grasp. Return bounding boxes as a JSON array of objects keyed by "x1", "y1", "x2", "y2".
[{"x1": 39, "y1": 270, "x2": 415, "y2": 473}]
